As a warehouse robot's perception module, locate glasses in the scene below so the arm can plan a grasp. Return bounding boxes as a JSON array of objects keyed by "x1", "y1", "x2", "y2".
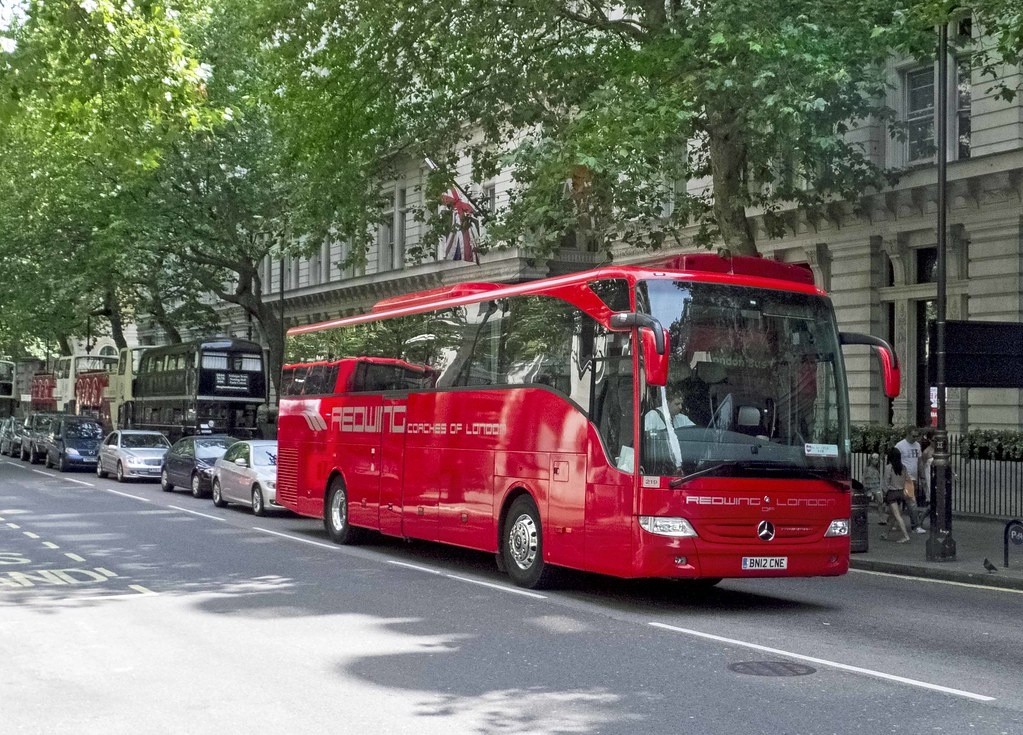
[{"x1": 913, "y1": 435, "x2": 919, "y2": 438}]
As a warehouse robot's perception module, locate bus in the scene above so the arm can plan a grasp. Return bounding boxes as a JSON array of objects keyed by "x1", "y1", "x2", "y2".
[
  {"x1": 135, "y1": 339, "x2": 270, "y2": 438},
  {"x1": 277, "y1": 254, "x2": 901, "y2": 588},
  {"x1": 279, "y1": 356, "x2": 436, "y2": 396}
]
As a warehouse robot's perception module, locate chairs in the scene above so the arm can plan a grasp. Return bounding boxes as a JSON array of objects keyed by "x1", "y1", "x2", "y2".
[{"x1": 288, "y1": 354, "x2": 727, "y2": 472}]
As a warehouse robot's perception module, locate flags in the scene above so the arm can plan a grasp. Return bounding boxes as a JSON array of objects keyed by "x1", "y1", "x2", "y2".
[{"x1": 423, "y1": 154, "x2": 481, "y2": 268}]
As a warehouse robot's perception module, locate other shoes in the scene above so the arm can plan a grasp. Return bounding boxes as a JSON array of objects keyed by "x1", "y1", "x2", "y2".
[
  {"x1": 896, "y1": 537, "x2": 911, "y2": 544},
  {"x1": 879, "y1": 533, "x2": 887, "y2": 540},
  {"x1": 878, "y1": 520, "x2": 887, "y2": 525},
  {"x1": 911, "y1": 527, "x2": 927, "y2": 534},
  {"x1": 892, "y1": 527, "x2": 896, "y2": 530}
]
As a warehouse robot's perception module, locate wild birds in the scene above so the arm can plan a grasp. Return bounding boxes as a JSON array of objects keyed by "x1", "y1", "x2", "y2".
[{"x1": 983, "y1": 558, "x2": 997, "y2": 573}]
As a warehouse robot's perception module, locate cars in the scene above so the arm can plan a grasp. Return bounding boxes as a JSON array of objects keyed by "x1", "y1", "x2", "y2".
[
  {"x1": 210, "y1": 440, "x2": 294, "y2": 516},
  {"x1": 161, "y1": 435, "x2": 242, "y2": 498},
  {"x1": 96, "y1": 429, "x2": 172, "y2": 482},
  {"x1": 0, "y1": 414, "x2": 105, "y2": 472}
]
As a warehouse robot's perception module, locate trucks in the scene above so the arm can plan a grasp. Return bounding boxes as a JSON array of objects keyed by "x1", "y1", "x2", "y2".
[{"x1": 0, "y1": 346, "x2": 168, "y2": 429}]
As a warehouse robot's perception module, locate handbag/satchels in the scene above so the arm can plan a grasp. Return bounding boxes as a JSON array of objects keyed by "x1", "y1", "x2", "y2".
[{"x1": 902, "y1": 466, "x2": 915, "y2": 500}]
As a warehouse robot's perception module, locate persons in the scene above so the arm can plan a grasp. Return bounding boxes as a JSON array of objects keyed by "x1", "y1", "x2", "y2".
[
  {"x1": 642, "y1": 389, "x2": 696, "y2": 432},
  {"x1": 864, "y1": 425, "x2": 958, "y2": 543}
]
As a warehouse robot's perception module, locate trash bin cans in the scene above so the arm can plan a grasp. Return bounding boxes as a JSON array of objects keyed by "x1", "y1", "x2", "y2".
[{"x1": 851, "y1": 478, "x2": 870, "y2": 554}]
